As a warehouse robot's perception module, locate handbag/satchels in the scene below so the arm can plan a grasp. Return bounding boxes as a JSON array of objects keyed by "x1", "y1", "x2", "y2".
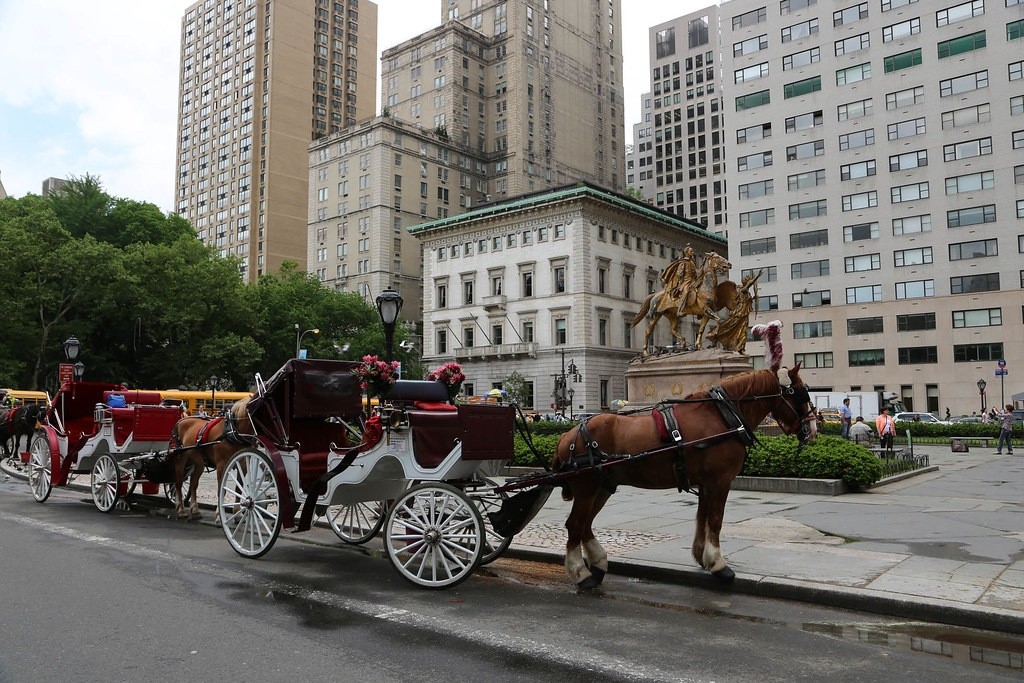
[
  {"x1": 361, "y1": 416, "x2": 384, "y2": 449},
  {"x1": 108, "y1": 393, "x2": 126, "y2": 408}
]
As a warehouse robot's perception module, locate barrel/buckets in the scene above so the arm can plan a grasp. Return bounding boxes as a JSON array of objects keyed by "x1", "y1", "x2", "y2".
[
  {"x1": 20, "y1": 451, "x2": 31, "y2": 462},
  {"x1": 111, "y1": 470, "x2": 130, "y2": 496},
  {"x1": 142, "y1": 481, "x2": 160, "y2": 494}
]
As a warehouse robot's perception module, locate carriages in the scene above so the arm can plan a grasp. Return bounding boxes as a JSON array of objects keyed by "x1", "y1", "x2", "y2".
[
  {"x1": 20, "y1": 379, "x2": 257, "y2": 528},
  {"x1": 0, "y1": 402, "x2": 49, "y2": 462},
  {"x1": 246, "y1": 319, "x2": 818, "y2": 590}
]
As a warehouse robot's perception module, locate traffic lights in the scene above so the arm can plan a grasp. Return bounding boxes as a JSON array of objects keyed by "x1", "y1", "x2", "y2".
[
  {"x1": 573, "y1": 374, "x2": 577, "y2": 383},
  {"x1": 578, "y1": 374, "x2": 583, "y2": 382},
  {"x1": 568, "y1": 364, "x2": 572, "y2": 374},
  {"x1": 572, "y1": 364, "x2": 577, "y2": 374}
]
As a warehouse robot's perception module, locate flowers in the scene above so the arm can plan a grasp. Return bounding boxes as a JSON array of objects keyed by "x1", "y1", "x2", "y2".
[
  {"x1": 2, "y1": 394, "x2": 19, "y2": 406},
  {"x1": 427, "y1": 363, "x2": 466, "y2": 398},
  {"x1": 351, "y1": 355, "x2": 400, "y2": 397}
]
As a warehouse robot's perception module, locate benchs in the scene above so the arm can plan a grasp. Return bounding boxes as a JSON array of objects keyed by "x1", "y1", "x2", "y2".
[
  {"x1": 950, "y1": 436, "x2": 995, "y2": 447},
  {"x1": 100, "y1": 390, "x2": 163, "y2": 419},
  {"x1": 394, "y1": 378, "x2": 459, "y2": 420},
  {"x1": 868, "y1": 447, "x2": 904, "y2": 459}
]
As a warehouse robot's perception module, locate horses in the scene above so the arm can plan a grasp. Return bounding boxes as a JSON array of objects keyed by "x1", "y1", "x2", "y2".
[
  {"x1": 170, "y1": 394, "x2": 254, "y2": 528},
  {"x1": 0, "y1": 400, "x2": 49, "y2": 461},
  {"x1": 553, "y1": 360, "x2": 818, "y2": 590},
  {"x1": 631, "y1": 251, "x2": 732, "y2": 357}
]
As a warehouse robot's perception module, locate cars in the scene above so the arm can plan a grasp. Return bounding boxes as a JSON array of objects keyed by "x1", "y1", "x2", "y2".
[
  {"x1": 1012, "y1": 410, "x2": 1024, "y2": 429},
  {"x1": 821, "y1": 413, "x2": 843, "y2": 425},
  {"x1": 948, "y1": 416, "x2": 998, "y2": 424}
]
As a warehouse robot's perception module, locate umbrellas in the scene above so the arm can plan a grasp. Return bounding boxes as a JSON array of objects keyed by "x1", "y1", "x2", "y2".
[
  {"x1": 610, "y1": 399, "x2": 628, "y2": 408},
  {"x1": 483, "y1": 388, "x2": 507, "y2": 398}
]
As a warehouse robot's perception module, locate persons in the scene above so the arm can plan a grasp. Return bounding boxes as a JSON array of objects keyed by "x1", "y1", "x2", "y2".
[
  {"x1": 195, "y1": 405, "x2": 209, "y2": 416},
  {"x1": 848, "y1": 416, "x2": 875, "y2": 448},
  {"x1": 912, "y1": 414, "x2": 921, "y2": 422},
  {"x1": 525, "y1": 411, "x2": 551, "y2": 425},
  {"x1": 706, "y1": 268, "x2": 765, "y2": 356},
  {"x1": 673, "y1": 245, "x2": 700, "y2": 317},
  {"x1": 840, "y1": 398, "x2": 852, "y2": 439},
  {"x1": 993, "y1": 404, "x2": 1015, "y2": 455},
  {"x1": 875, "y1": 407, "x2": 897, "y2": 458},
  {"x1": 981, "y1": 408, "x2": 988, "y2": 424}
]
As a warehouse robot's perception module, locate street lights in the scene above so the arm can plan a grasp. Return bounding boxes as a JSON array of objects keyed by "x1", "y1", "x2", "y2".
[
  {"x1": 568, "y1": 387, "x2": 576, "y2": 422},
  {"x1": 209, "y1": 375, "x2": 218, "y2": 416},
  {"x1": 976, "y1": 379, "x2": 987, "y2": 415},
  {"x1": 74, "y1": 360, "x2": 86, "y2": 383},
  {"x1": 376, "y1": 286, "x2": 404, "y2": 364},
  {"x1": 294, "y1": 323, "x2": 320, "y2": 360}
]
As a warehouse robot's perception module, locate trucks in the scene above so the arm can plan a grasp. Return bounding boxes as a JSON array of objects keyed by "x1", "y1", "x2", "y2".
[{"x1": 807, "y1": 390, "x2": 908, "y2": 423}]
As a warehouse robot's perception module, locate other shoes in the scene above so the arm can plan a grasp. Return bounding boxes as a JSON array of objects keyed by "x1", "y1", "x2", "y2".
[
  {"x1": 995, "y1": 452, "x2": 1002, "y2": 454},
  {"x1": 1006, "y1": 452, "x2": 1013, "y2": 455}
]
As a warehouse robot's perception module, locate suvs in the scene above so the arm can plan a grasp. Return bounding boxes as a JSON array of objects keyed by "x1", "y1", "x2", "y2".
[{"x1": 891, "y1": 412, "x2": 954, "y2": 427}]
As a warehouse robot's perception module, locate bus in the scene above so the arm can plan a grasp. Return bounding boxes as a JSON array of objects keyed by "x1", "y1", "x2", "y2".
[
  {"x1": 360, "y1": 397, "x2": 380, "y2": 420},
  {"x1": 0, "y1": 388, "x2": 48, "y2": 431},
  {"x1": 126, "y1": 389, "x2": 254, "y2": 423}
]
